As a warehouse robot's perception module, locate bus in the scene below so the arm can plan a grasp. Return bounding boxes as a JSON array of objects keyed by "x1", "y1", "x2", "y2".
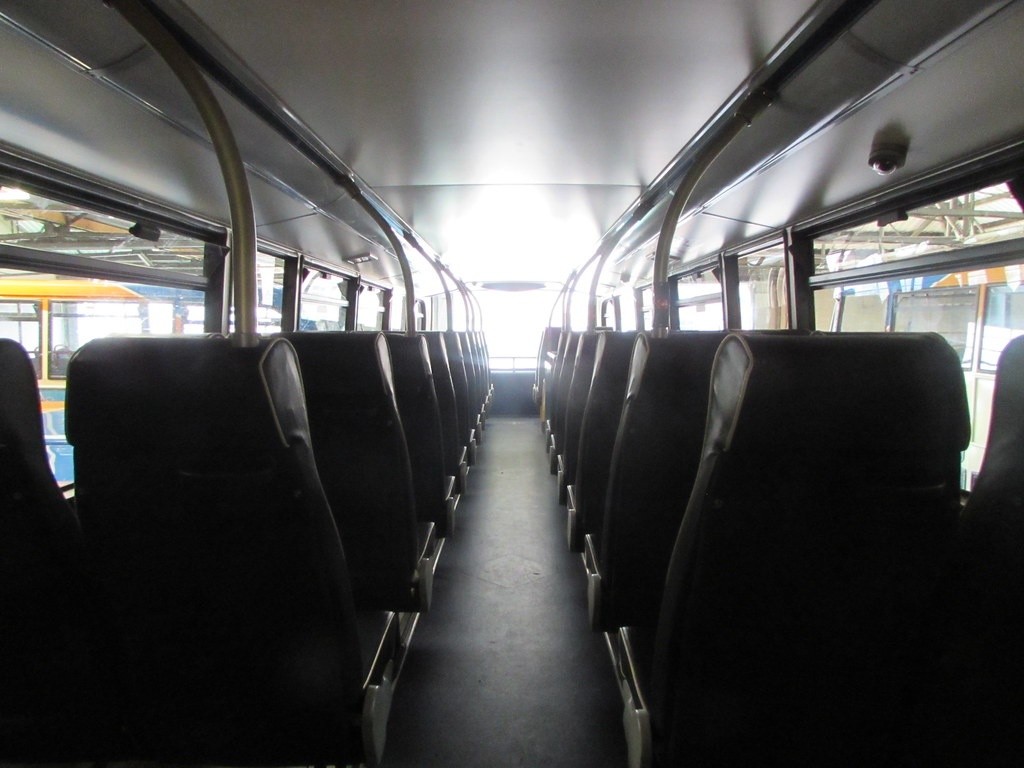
[{"x1": 1, "y1": 279, "x2": 286, "y2": 504}]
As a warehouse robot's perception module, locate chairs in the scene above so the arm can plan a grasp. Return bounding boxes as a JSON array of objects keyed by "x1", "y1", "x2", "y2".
[{"x1": 0, "y1": 327, "x2": 1024, "y2": 768}]
